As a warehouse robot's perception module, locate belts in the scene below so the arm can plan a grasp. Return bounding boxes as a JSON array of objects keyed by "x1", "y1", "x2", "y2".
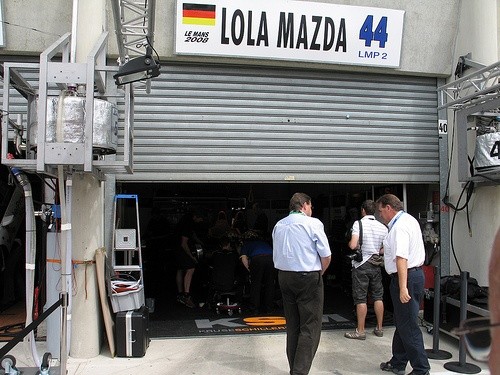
[
  {"x1": 390, "y1": 266, "x2": 422, "y2": 278},
  {"x1": 253, "y1": 254, "x2": 273, "y2": 257}
]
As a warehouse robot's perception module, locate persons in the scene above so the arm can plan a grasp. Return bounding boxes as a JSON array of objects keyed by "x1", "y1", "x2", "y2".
[
  {"x1": 210, "y1": 236, "x2": 237, "y2": 296},
  {"x1": 239, "y1": 231, "x2": 274, "y2": 315},
  {"x1": 272, "y1": 193, "x2": 331, "y2": 375},
  {"x1": 174, "y1": 207, "x2": 206, "y2": 306},
  {"x1": 486, "y1": 226, "x2": 500, "y2": 375},
  {"x1": 375, "y1": 194, "x2": 431, "y2": 375},
  {"x1": 252, "y1": 203, "x2": 268, "y2": 241},
  {"x1": 345, "y1": 200, "x2": 389, "y2": 340}
]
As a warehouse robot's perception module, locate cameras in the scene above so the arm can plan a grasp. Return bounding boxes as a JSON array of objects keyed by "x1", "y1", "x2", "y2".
[{"x1": 345, "y1": 250, "x2": 362, "y2": 262}]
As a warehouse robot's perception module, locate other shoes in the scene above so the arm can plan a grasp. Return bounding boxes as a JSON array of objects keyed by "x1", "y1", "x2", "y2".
[
  {"x1": 380, "y1": 361, "x2": 405, "y2": 374},
  {"x1": 374, "y1": 325, "x2": 383, "y2": 336},
  {"x1": 344, "y1": 328, "x2": 366, "y2": 339},
  {"x1": 176, "y1": 293, "x2": 195, "y2": 307},
  {"x1": 407, "y1": 371, "x2": 430, "y2": 375}
]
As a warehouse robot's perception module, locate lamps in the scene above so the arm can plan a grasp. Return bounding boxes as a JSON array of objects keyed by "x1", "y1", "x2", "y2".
[{"x1": 113, "y1": 53, "x2": 162, "y2": 87}]
